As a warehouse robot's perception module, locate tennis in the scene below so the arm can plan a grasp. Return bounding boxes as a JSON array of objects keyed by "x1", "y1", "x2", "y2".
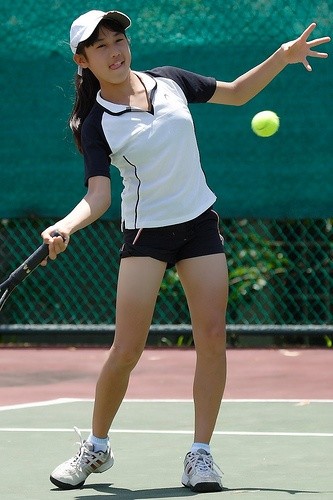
[{"x1": 251, "y1": 110, "x2": 279, "y2": 137}]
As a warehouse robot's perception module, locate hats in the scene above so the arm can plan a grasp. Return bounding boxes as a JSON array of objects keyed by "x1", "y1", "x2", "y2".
[{"x1": 69, "y1": 9, "x2": 132, "y2": 55}]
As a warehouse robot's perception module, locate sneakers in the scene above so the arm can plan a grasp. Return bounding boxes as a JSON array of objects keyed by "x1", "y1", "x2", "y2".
[
  {"x1": 181, "y1": 448, "x2": 224, "y2": 492},
  {"x1": 49, "y1": 425, "x2": 114, "y2": 488}
]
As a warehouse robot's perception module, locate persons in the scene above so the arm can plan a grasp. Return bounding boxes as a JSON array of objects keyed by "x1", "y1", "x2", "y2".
[{"x1": 41, "y1": 10, "x2": 331, "y2": 493}]
